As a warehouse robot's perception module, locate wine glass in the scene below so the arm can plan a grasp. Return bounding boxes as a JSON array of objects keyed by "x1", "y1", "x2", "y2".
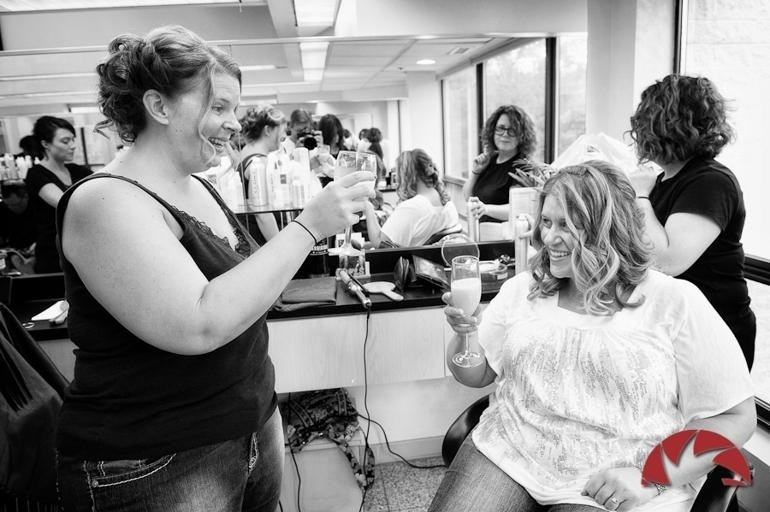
[
  {"x1": 450, "y1": 254, "x2": 483, "y2": 369},
  {"x1": 326, "y1": 149, "x2": 376, "y2": 258}
]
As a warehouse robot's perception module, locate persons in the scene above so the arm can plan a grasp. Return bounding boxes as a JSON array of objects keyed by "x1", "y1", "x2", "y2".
[
  {"x1": 628, "y1": 75, "x2": 756, "y2": 374},
  {"x1": 45, "y1": 27, "x2": 379, "y2": 512},
  {"x1": 16, "y1": 94, "x2": 549, "y2": 275},
  {"x1": 428, "y1": 160, "x2": 756, "y2": 512}
]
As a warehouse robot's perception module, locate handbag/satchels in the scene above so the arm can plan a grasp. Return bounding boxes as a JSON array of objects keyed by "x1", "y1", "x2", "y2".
[{"x1": 279, "y1": 388, "x2": 360, "y2": 445}]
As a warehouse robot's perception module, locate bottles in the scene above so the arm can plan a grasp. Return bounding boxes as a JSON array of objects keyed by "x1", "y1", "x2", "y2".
[
  {"x1": 290, "y1": 176, "x2": 310, "y2": 209},
  {"x1": 250, "y1": 157, "x2": 268, "y2": 206},
  {"x1": 0, "y1": 153, "x2": 41, "y2": 184}
]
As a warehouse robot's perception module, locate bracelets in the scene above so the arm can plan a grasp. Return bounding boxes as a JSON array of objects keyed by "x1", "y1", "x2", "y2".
[
  {"x1": 292, "y1": 219, "x2": 316, "y2": 244},
  {"x1": 636, "y1": 457, "x2": 669, "y2": 495},
  {"x1": 635, "y1": 195, "x2": 654, "y2": 200}
]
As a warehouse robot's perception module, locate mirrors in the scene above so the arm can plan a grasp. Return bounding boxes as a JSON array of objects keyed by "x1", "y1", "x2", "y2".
[
  {"x1": 1, "y1": 96, "x2": 402, "y2": 215},
  {"x1": 1, "y1": 31, "x2": 557, "y2": 279}
]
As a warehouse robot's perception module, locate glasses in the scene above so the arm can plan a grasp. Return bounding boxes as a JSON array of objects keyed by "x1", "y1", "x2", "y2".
[{"x1": 493, "y1": 125, "x2": 517, "y2": 138}]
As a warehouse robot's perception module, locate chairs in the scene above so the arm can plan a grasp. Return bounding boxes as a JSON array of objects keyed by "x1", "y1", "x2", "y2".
[
  {"x1": 440, "y1": 390, "x2": 747, "y2": 512},
  {"x1": 422, "y1": 220, "x2": 463, "y2": 246}
]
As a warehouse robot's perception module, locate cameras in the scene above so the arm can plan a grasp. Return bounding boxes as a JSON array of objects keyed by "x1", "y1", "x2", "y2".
[{"x1": 297, "y1": 127, "x2": 317, "y2": 150}]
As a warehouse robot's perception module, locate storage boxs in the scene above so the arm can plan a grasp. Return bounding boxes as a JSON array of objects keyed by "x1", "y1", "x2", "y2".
[{"x1": 278, "y1": 411, "x2": 367, "y2": 512}]
